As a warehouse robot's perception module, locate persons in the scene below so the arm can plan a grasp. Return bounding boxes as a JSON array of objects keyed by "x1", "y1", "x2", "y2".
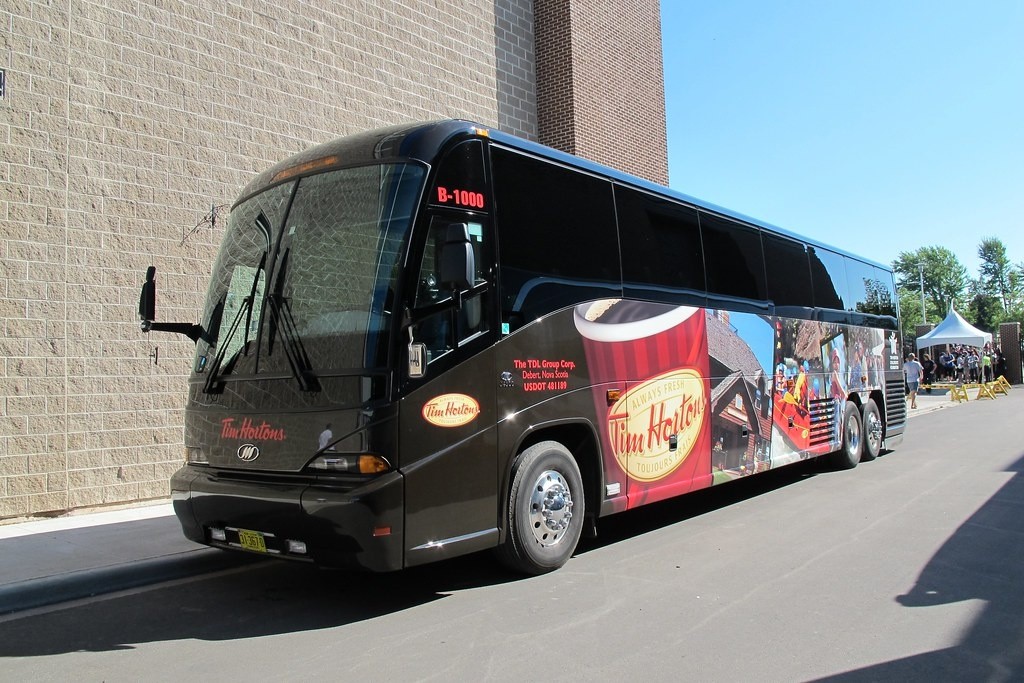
[
  {"x1": 829, "y1": 349, "x2": 845, "y2": 401},
  {"x1": 939, "y1": 344, "x2": 1006, "y2": 384},
  {"x1": 922, "y1": 354, "x2": 937, "y2": 395},
  {"x1": 316, "y1": 422, "x2": 334, "y2": 452},
  {"x1": 903, "y1": 353, "x2": 924, "y2": 408},
  {"x1": 793, "y1": 361, "x2": 810, "y2": 416},
  {"x1": 782, "y1": 369, "x2": 792, "y2": 397},
  {"x1": 776, "y1": 363, "x2": 784, "y2": 395},
  {"x1": 808, "y1": 378, "x2": 821, "y2": 421}
]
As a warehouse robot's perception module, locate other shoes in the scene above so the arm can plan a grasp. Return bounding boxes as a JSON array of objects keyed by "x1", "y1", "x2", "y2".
[{"x1": 911, "y1": 405, "x2": 917, "y2": 409}]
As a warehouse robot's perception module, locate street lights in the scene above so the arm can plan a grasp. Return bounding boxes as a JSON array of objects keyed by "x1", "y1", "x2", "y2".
[{"x1": 915, "y1": 261, "x2": 929, "y2": 325}]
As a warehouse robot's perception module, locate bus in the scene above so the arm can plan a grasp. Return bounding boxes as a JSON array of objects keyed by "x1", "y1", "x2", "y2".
[{"x1": 138, "y1": 118, "x2": 905, "y2": 578}]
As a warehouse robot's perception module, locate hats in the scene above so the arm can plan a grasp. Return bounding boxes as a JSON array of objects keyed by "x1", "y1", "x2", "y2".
[{"x1": 832, "y1": 348, "x2": 841, "y2": 363}]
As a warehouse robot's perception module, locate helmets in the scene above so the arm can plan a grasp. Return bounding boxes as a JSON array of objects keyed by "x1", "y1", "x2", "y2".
[{"x1": 778, "y1": 360, "x2": 820, "y2": 398}]
